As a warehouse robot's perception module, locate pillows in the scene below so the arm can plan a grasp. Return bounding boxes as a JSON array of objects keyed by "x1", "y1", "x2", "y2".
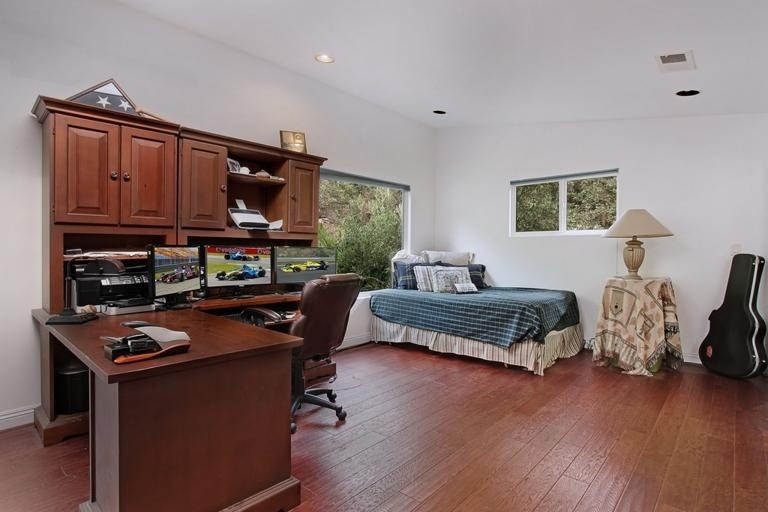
[{"x1": 391, "y1": 249, "x2": 492, "y2": 294}]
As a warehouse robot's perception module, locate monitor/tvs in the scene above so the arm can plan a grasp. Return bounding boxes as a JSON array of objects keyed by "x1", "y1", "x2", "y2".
[
  {"x1": 205, "y1": 243, "x2": 272, "y2": 299},
  {"x1": 147, "y1": 243, "x2": 203, "y2": 308},
  {"x1": 273, "y1": 244, "x2": 337, "y2": 295}
]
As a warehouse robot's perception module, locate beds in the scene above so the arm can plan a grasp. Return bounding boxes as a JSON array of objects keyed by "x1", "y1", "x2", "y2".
[{"x1": 370, "y1": 288, "x2": 586, "y2": 377}]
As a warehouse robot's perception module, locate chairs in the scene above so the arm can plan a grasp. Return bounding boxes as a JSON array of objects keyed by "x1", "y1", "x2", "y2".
[{"x1": 240, "y1": 272, "x2": 365, "y2": 434}]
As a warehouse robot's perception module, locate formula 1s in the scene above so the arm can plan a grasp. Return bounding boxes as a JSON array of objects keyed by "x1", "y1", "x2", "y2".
[
  {"x1": 224, "y1": 250, "x2": 258, "y2": 260},
  {"x1": 280, "y1": 259, "x2": 329, "y2": 272},
  {"x1": 158, "y1": 265, "x2": 196, "y2": 284},
  {"x1": 215, "y1": 263, "x2": 265, "y2": 281}
]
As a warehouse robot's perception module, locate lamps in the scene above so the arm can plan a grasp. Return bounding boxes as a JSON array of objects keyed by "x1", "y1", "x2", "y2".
[{"x1": 601, "y1": 209, "x2": 675, "y2": 279}]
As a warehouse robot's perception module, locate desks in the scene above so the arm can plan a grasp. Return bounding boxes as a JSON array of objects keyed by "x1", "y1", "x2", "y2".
[
  {"x1": 193, "y1": 292, "x2": 337, "y2": 382},
  {"x1": 592, "y1": 275, "x2": 684, "y2": 377},
  {"x1": 31, "y1": 308, "x2": 305, "y2": 512}
]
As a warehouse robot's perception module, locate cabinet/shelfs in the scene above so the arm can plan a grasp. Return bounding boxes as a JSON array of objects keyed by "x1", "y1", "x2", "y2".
[
  {"x1": 32, "y1": 95, "x2": 180, "y2": 314},
  {"x1": 179, "y1": 125, "x2": 328, "y2": 314}
]
